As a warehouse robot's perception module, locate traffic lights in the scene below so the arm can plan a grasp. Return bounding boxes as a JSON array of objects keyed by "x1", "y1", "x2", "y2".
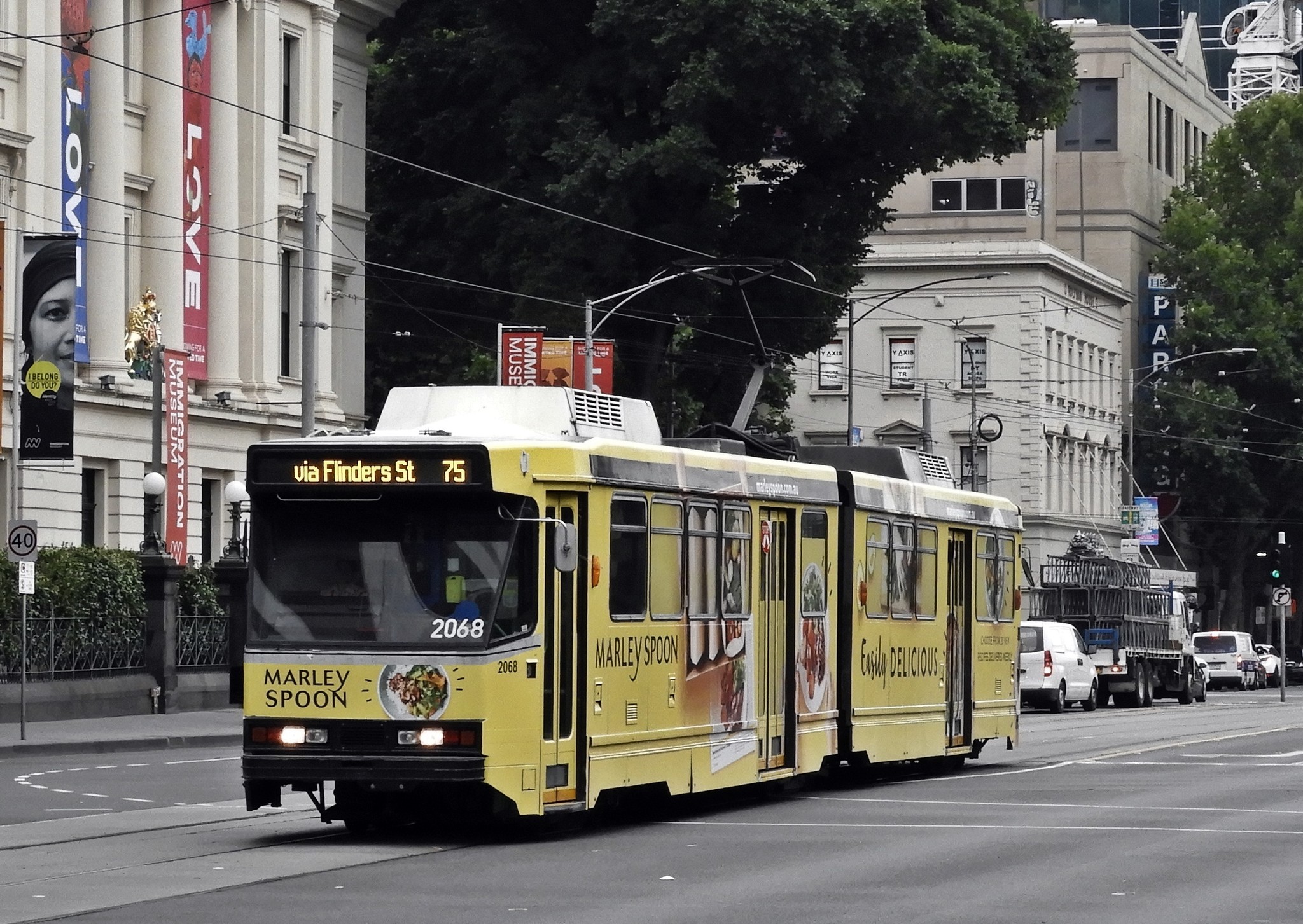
[{"x1": 1270, "y1": 549, "x2": 1284, "y2": 583}]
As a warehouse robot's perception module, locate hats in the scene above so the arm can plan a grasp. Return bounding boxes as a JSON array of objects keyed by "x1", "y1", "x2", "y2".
[{"x1": 22, "y1": 240, "x2": 76, "y2": 347}]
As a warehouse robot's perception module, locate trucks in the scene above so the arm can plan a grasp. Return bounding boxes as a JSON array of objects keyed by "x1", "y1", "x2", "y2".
[{"x1": 1026, "y1": 552, "x2": 1199, "y2": 709}]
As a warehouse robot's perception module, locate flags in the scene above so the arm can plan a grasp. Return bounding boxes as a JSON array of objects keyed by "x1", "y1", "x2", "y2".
[
  {"x1": 163, "y1": 344, "x2": 193, "y2": 574},
  {"x1": 572, "y1": 342, "x2": 615, "y2": 395},
  {"x1": 501, "y1": 330, "x2": 544, "y2": 388},
  {"x1": 540, "y1": 339, "x2": 574, "y2": 389},
  {"x1": 20, "y1": 234, "x2": 77, "y2": 463},
  {"x1": 1131, "y1": 496, "x2": 1160, "y2": 547}
]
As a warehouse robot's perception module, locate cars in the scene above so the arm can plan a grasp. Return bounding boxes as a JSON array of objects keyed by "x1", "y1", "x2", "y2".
[{"x1": 1259, "y1": 663, "x2": 1268, "y2": 690}]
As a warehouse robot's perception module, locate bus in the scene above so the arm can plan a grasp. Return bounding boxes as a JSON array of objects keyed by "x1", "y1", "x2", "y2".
[{"x1": 240, "y1": 382, "x2": 1025, "y2": 841}]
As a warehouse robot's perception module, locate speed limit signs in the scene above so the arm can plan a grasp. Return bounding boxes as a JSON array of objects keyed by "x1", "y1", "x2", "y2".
[{"x1": 8, "y1": 519, "x2": 38, "y2": 563}]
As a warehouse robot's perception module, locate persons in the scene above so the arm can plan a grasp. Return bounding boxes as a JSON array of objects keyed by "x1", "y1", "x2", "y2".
[
  {"x1": 126, "y1": 285, "x2": 164, "y2": 366},
  {"x1": 22, "y1": 240, "x2": 76, "y2": 420}
]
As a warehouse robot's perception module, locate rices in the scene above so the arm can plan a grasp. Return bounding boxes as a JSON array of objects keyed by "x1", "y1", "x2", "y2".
[{"x1": 386, "y1": 664, "x2": 413, "y2": 714}]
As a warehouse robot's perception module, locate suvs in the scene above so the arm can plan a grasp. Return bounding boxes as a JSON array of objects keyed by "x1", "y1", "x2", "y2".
[
  {"x1": 1276, "y1": 645, "x2": 1303, "y2": 683},
  {"x1": 1191, "y1": 632, "x2": 1260, "y2": 691},
  {"x1": 1255, "y1": 643, "x2": 1288, "y2": 688},
  {"x1": 1017, "y1": 619, "x2": 1100, "y2": 713}
]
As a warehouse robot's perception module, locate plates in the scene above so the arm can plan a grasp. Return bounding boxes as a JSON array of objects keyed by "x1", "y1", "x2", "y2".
[
  {"x1": 797, "y1": 564, "x2": 827, "y2": 712},
  {"x1": 377, "y1": 664, "x2": 451, "y2": 720}
]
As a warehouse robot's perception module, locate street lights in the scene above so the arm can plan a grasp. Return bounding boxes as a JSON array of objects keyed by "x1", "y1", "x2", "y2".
[
  {"x1": 1129, "y1": 348, "x2": 1258, "y2": 539},
  {"x1": 222, "y1": 481, "x2": 249, "y2": 560},
  {"x1": 848, "y1": 270, "x2": 1011, "y2": 447},
  {"x1": 953, "y1": 336, "x2": 979, "y2": 494},
  {"x1": 583, "y1": 256, "x2": 815, "y2": 393},
  {"x1": 140, "y1": 472, "x2": 170, "y2": 555}
]
